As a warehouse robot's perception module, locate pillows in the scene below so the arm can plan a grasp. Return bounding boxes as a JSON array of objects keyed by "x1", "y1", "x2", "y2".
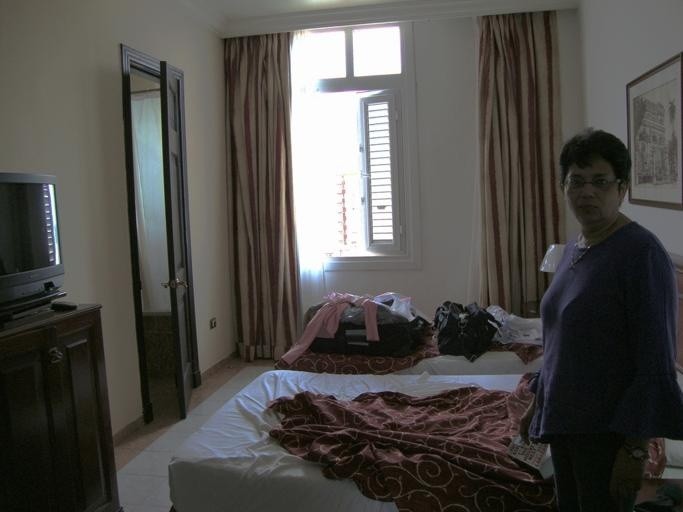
[{"x1": 664, "y1": 371, "x2": 683, "y2": 469}]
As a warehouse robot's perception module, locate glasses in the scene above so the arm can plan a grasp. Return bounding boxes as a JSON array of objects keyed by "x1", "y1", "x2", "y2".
[{"x1": 560, "y1": 171, "x2": 626, "y2": 192}]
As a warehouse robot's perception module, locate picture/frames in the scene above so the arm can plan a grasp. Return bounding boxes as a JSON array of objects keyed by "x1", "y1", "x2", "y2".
[{"x1": 626, "y1": 50, "x2": 683, "y2": 212}]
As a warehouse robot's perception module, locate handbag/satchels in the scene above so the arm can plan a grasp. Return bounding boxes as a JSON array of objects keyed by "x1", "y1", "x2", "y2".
[{"x1": 433, "y1": 300, "x2": 501, "y2": 362}]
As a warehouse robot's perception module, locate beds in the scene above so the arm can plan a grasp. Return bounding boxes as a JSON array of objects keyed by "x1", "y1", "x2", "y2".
[
  {"x1": 274, "y1": 317, "x2": 543, "y2": 375},
  {"x1": 165, "y1": 370, "x2": 683, "y2": 512}
]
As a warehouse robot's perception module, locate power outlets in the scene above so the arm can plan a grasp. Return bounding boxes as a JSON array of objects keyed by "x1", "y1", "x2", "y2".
[{"x1": 210, "y1": 318, "x2": 217, "y2": 329}]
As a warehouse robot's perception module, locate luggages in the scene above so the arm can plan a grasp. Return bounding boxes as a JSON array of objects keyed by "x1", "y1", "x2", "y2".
[{"x1": 303, "y1": 297, "x2": 432, "y2": 357}]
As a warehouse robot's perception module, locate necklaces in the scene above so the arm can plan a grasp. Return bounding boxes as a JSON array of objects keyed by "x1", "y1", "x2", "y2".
[{"x1": 565, "y1": 212, "x2": 623, "y2": 272}]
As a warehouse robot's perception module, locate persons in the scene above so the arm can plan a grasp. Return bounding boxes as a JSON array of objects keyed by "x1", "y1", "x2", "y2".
[{"x1": 516, "y1": 123, "x2": 681, "y2": 509}]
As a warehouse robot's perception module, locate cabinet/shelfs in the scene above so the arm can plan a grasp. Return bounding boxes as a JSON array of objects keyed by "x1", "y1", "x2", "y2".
[{"x1": 0, "y1": 303, "x2": 124, "y2": 512}]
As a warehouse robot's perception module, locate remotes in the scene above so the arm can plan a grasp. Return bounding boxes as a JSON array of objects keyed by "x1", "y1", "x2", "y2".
[{"x1": 51, "y1": 301, "x2": 77, "y2": 310}]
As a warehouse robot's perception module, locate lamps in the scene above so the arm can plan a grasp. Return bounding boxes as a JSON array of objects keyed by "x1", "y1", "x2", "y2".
[{"x1": 537, "y1": 244, "x2": 570, "y2": 274}]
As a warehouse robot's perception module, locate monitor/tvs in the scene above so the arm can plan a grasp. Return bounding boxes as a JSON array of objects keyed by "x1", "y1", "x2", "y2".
[{"x1": 0, "y1": 171, "x2": 67, "y2": 319}]
